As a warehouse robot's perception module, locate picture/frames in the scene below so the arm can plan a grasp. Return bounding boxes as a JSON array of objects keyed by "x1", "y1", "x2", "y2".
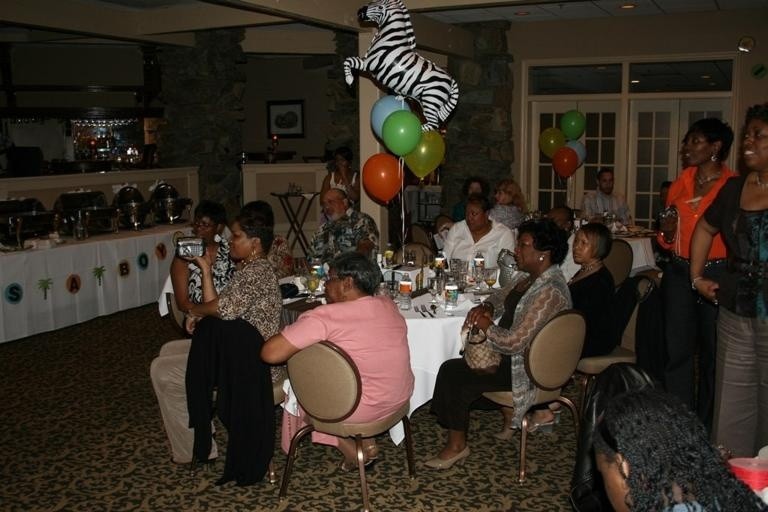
[{"x1": 265, "y1": 98, "x2": 308, "y2": 140}]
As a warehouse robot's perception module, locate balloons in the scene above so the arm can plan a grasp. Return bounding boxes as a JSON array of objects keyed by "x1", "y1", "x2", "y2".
[
  {"x1": 344, "y1": 1, "x2": 459, "y2": 207},
  {"x1": 537, "y1": 109, "x2": 588, "y2": 180}
]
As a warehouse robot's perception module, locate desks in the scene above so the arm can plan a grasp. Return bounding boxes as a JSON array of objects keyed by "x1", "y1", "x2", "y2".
[
  {"x1": 270, "y1": 191, "x2": 320, "y2": 257},
  {"x1": 0, "y1": 218, "x2": 197, "y2": 345}
]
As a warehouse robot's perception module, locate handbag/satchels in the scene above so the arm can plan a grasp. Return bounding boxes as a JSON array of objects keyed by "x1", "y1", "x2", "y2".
[
  {"x1": 655, "y1": 207, "x2": 678, "y2": 243},
  {"x1": 463, "y1": 327, "x2": 503, "y2": 369},
  {"x1": 497, "y1": 249, "x2": 516, "y2": 288}
]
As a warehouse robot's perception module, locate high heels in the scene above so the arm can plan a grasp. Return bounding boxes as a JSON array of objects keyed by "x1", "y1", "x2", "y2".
[
  {"x1": 494, "y1": 406, "x2": 562, "y2": 440},
  {"x1": 424, "y1": 446, "x2": 471, "y2": 471}
]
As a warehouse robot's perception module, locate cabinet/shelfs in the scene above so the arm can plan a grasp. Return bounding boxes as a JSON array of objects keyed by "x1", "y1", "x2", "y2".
[{"x1": 415, "y1": 185, "x2": 443, "y2": 223}]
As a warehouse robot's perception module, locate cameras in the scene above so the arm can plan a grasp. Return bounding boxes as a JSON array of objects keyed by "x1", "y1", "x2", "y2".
[{"x1": 175, "y1": 237, "x2": 205, "y2": 260}]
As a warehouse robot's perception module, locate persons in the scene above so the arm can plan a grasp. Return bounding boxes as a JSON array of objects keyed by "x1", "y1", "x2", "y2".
[
  {"x1": 150, "y1": 190, "x2": 414, "y2": 487},
  {"x1": 421, "y1": 167, "x2": 636, "y2": 470},
  {"x1": 591, "y1": 390, "x2": 768, "y2": 512},
  {"x1": 319, "y1": 148, "x2": 359, "y2": 226},
  {"x1": 655, "y1": 102, "x2": 768, "y2": 459}
]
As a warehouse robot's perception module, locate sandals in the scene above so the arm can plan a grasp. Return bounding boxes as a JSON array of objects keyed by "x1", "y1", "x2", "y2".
[{"x1": 338, "y1": 445, "x2": 378, "y2": 473}]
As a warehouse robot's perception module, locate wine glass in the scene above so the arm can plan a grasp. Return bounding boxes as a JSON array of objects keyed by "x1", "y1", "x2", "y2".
[
  {"x1": 297, "y1": 262, "x2": 323, "y2": 302},
  {"x1": 606, "y1": 211, "x2": 641, "y2": 236},
  {"x1": 427, "y1": 247, "x2": 498, "y2": 306}
]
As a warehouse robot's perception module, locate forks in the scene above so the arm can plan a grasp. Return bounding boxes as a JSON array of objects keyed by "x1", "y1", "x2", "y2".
[{"x1": 415, "y1": 303, "x2": 438, "y2": 320}]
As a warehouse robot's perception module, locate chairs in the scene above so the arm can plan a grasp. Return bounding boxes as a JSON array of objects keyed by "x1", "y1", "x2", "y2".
[
  {"x1": 165, "y1": 273, "x2": 187, "y2": 334},
  {"x1": 193, "y1": 315, "x2": 290, "y2": 484},
  {"x1": 392, "y1": 214, "x2": 455, "y2": 269},
  {"x1": 279, "y1": 339, "x2": 416, "y2": 512}
]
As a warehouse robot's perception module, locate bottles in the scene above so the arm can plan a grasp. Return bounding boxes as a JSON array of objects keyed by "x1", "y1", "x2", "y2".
[
  {"x1": 383, "y1": 242, "x2": 394, "y2": 270},
  {"x1": 398, "y1": 272, "x2": 413, "y2": 311},
  {"x1": 443, "y1": 276, "x2": 459, "y2": 311}
]
